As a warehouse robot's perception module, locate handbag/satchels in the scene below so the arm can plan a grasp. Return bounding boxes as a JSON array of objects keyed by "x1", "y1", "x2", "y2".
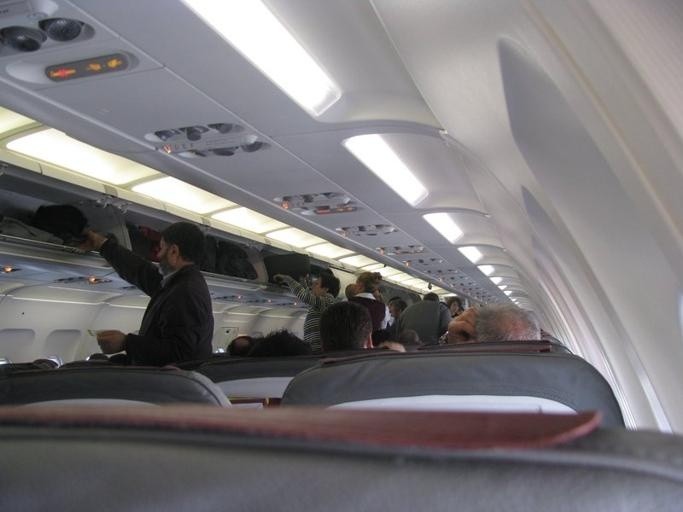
[
  {"x1": 202, "y1": 237, "x2": 256, "y2": 280},
  {"x1": 1, "y1": 204, "x2": 86, "y2": 245},
  {"x1": 267, "y1": 252, "x2": 308, "y2": 284},
  {"x1": 129, "y1": 224, "x2": 161, "y2": 260}
]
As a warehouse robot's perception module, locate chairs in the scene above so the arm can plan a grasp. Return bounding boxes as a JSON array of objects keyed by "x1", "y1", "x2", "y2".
[{"x1": 0, "y1": 331, "x2": 683, "y2": 512}]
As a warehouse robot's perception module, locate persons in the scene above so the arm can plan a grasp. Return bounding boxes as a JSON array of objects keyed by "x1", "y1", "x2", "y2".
[
  {"x1": 226, "y1": 332, "x2": 310, "y2": 362},
  {"x1": 386, "y1": 292, "x2": 543, "y2": 343},
  {"x1": 272, "y1": 270, "x2": 342, "y2": 355},
  {"x1": 346, "y1": 270, "x2": 385, "y2": 346},
  {"x1": 74, "y1": 221, "x2": 216, "y2": 371}
]
